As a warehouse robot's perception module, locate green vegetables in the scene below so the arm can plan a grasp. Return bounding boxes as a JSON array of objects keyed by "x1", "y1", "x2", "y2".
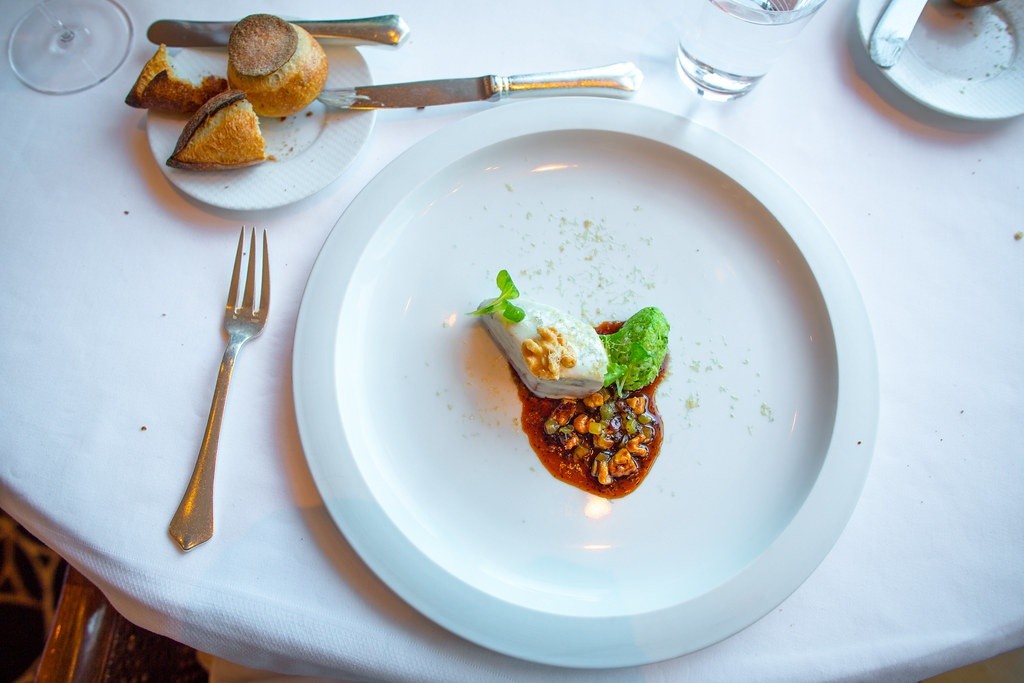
[
  {"x1": 466, "y1": 270, "x2": 526, "y2": 323},
  {"x1": 604, "y1": 343, "x2": 650, "y2": 398}
]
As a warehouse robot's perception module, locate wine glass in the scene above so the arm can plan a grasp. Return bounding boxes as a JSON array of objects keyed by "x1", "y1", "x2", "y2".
[{"x1": 0, "y1": 0, "x2": 134, "y2": 95}]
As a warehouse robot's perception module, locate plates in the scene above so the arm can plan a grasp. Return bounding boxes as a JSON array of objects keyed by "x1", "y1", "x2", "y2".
[
  {"x1": 146, "y1": 47, "x2": 376, "y2": 212},
  {"x1": 855, "y1": 0, "x2": 1023, "y2": 122},
  {"x1": 290, "y1": 96, "x2": 879, "y2": 672}
]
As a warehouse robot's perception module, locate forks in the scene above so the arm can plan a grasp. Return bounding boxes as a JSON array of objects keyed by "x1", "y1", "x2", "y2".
[{"x1": 166, "y1": 226, "x2": 271, "y2": 552}]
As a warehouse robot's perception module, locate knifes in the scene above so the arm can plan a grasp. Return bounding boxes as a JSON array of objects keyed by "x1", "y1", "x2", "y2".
[
  {"x1": 146, "y1": 14, "x2": 411, "y2": 48},
  {"x1": 869, "y1": 0, "x2": 928, "y2": 67},
  {"x1": 315, "y1": 62, "x2": 646, "y2": 111}
]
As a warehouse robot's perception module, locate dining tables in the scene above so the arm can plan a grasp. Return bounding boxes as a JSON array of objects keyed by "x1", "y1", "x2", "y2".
[{"x1": 0, "y1": 0, "x2": 1024, "y2": 683}]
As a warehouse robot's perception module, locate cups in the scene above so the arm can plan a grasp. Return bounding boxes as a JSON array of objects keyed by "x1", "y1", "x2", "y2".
[{"x1": 675, "y1": 0, "x2": 827, "y2": 101}]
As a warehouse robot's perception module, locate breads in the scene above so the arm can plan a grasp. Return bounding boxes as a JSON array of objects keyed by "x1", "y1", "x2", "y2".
[{"x1": 125, "y1": 13, "x2": 329, "y2": 170}]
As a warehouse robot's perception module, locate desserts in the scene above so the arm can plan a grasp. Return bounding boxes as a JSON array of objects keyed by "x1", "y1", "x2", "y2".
[{"x1": 476, "y1": 297, "x2": 609, "y2": 398}]
